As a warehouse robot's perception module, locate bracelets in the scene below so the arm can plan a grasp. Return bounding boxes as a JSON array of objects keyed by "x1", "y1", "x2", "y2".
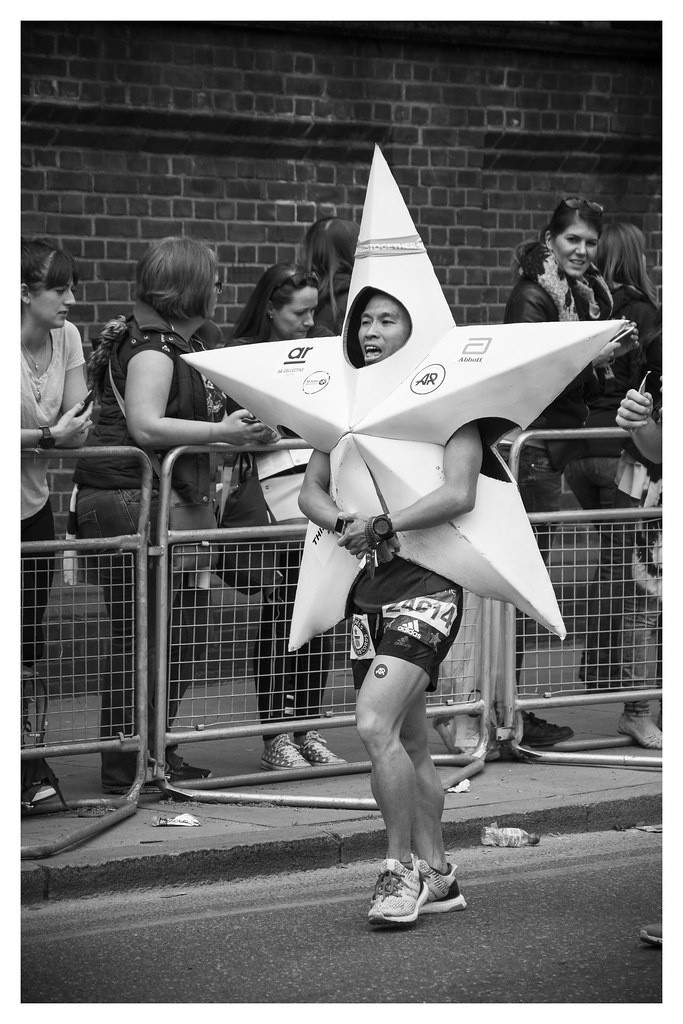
[{"x1": 359, "y1": 516, "x2": 381, "y2": 579}]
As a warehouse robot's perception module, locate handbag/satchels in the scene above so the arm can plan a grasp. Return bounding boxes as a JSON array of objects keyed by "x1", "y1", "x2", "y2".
[
  {"x1": 167, "y1": 488, "x2": 220, "y2": 571},
  {"x1": 212, "y1": 452, "x2": 279, "y2": 595}
]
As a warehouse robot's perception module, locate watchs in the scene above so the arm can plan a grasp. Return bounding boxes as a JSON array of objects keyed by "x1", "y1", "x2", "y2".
[
  {"x1": 334, "y1": 516, "x2": 347, "y2": 537},
  {"x1": 37, "y1": 426, "x2": 56, "y2": 451},
  {"x1": 371, "y1": 514, "x2": 395, "y2": 540}
]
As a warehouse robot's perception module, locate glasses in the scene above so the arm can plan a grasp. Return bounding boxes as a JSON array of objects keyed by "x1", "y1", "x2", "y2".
[
  {"x1": 213, "y1": 280, "x2": 222, "y2": 293},
  {"x1": 554, "y1": 196, "x2": 603, "y2": 217},
  {"x1": 272, "y1": 270, "x2": 321, "y2": 298}
]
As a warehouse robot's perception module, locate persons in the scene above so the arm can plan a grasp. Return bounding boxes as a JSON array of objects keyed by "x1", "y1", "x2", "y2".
[
  {"x1": 60, "y1": 236, "x2": 280, "y2": 794},
  {"x1": 296, "y1": 276, "x2": 483, "y2": 923},
  {"x1": 481, "y1": 195, "x2": 662, "y2": 749},
  {"x1": 295, "y1": 217, "x2": 359, "y2": 338},
  {"x1": 21, "y1": 238, "x2": 95, "y2": 806},
  {"x1": 230, "y1": 265, "x2": 350, "y2": 771}
]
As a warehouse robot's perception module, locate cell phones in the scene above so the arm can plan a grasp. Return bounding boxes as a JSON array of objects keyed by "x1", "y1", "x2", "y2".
[
  {"x1": 74, "y1": 390, "x2": 94, "y2": 417},
  {"x1": 610, "y1": 326, "x2": 635, "y2": 343}
]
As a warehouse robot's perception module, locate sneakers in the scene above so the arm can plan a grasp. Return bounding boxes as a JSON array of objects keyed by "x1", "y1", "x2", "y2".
[
  {"x1": 295, "y1": 731, "x2": 348, "y2": 765},
  {"x1": 367, "y1": 852, "x2": 429, "y2": 924},
  {"x1": 432, "y1": 710, "x2": 575, "y2": 761},
  {"x1": 103, "y1": 760, "x2": 212, "y2": 795},
  {"x1": 261, "y1": 734, "x2": 312, "y2": 772},
  {"x1": 370, "y1": 860, "x2": 467, "y2": 915},
  {"x1": 24, "y1": 781, "x2": 57, "y2": 803}
]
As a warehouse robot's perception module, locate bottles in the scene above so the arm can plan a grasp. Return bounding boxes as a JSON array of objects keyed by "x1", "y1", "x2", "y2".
[{"x1": 482, "y1": 826, "x2": 540, "y2": 847}]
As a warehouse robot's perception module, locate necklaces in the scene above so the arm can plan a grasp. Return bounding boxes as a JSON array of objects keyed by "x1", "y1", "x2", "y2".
[{"x1": 21, "y1": 339, "x2": 47, "y2": 402}]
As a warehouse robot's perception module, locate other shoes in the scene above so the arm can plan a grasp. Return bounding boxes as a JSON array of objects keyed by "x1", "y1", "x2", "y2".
[
  {"x1": 586, "y1": 680, "x2": 643, "y2": 694},
  {"x1": 639, "y1": 924, "x2": 662, "y2": 944},
  {"x1": 617, "y1": 712, "x2": 662, "y2": 750}
]
